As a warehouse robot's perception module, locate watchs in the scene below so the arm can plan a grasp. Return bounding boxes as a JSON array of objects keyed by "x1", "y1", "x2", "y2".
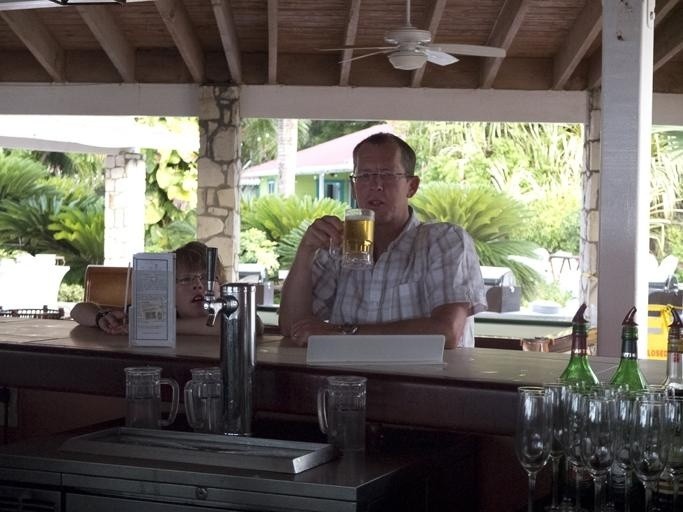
[
  {"x1": 96, "y1": 310, "x2": 110, "y2": 329},
  {"x1": 341, "y1": 323, "x2": 360, "y2": 335}
]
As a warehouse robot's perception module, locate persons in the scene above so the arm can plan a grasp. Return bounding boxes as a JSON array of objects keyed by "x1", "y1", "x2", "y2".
[
  {"x1": 70, "y1": 241, "x2": 265, "y2": 338},
  {"x1": 279, "y1": 133, "x2": 488, "y2": 349}
]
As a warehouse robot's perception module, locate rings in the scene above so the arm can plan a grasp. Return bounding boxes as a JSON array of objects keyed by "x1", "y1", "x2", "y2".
[{"x1": 293, "y1": 334, "x2": 298, "y2": 338}]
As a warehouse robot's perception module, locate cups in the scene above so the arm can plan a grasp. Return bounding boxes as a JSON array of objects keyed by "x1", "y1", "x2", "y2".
[
  {"x1": 317, "y1": 376, "x2": 367, "y2": 464},
  {"x1": 328, "y1": 208, "x2": 375, "y2": 271},
  {"x1": 184, "y1": 367, "x2": 224, "y2": 434},
  {"x1": 122, "y1": 367, "x2": 179, "y2": 429}
]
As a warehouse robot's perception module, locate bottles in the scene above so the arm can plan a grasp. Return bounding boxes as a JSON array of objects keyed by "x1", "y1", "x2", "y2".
[
  {"x1": 559, "y1": 323, "x2": 600, "y2": 384},
  {"x1": 609, "y1": 325, "x2": 649, "y2": 389},
  {"x1": 655, "y1": 326, "x2": 683, "y2": 401}
]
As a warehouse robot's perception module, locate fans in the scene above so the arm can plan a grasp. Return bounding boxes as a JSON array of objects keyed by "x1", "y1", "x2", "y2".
[{"x1": 316, "y1": 0, "x2": 506, "y2": 71}]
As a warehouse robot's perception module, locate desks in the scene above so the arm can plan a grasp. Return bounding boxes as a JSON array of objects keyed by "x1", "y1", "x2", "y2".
[
  {"x1": 0, "y1": 412, "x2": 482, "y2": 512},
  {"x1": 256, "y1": 305, "x2": 597, "y2": 352}
]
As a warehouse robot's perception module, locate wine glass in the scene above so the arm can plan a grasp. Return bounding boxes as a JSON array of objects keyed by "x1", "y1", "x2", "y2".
[{"x1": 516, "y1": 378, "x2": 683, "y2": 511}]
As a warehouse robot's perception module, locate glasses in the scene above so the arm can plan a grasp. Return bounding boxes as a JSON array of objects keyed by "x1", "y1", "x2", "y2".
[
  {"x1": 349, "y1": 170, "x2": 409, "y2": 183},
  {"x1": 176, "y1": 274, "x2": 219, "y2": 290}
]
